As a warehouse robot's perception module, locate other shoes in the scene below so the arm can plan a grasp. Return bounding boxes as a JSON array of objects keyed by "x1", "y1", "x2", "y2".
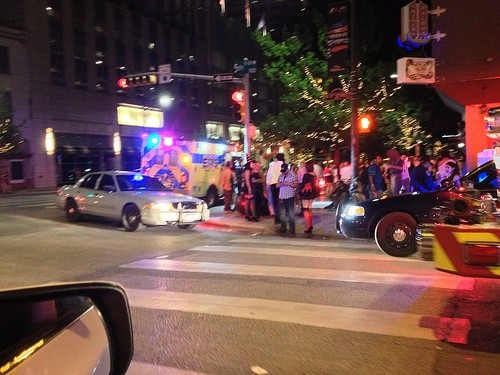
[
  {"x1": 245, "y1": 216, "x2": 259, "y2": 222},
  {"x1": 288, "y1": 229, "x2": 296, "y2": 234},
  {"x1": 304, "y1": 226, "x2": 313, "y2": 233},
  {"x1": 277, "y1": 228, "x2": 286, "y2": 232},
  {"x1": 224, "y1": 211, "x2": 231, "y2": 214}
]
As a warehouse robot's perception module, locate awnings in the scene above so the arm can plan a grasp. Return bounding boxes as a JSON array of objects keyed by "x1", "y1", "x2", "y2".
[{"x1": 54, "y1": 131, "x2": 143, "y2": 154}]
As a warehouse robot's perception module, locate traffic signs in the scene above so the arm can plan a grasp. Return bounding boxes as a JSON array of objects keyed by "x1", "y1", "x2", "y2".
[{"x1": 213, "y1": 72, "x2": 236, "y2": 82}]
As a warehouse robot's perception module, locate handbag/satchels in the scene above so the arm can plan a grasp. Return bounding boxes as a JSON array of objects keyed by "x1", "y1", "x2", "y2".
[
  {"x1": 297, "y1": 183, "x2": 314, "y2": 200},
  {"x1": 400, "y1": 164, "x2": 411, "y2": 183}
]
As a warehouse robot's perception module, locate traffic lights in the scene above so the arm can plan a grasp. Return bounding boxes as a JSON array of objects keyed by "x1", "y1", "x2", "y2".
[
  {"x1": 231, "y1": 90, "x2": 246, "y2": 123},
  {"x1": 118, "y1": 75, "x2": 157, "y2": 88},
  {"x1": 356, "y1": 112, "x2": 373, "y2": 133}
]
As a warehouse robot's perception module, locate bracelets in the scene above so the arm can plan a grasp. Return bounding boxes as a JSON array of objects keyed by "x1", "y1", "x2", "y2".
[{"x1": 371, "y1": 184, "x2": 374, "y2": 186}]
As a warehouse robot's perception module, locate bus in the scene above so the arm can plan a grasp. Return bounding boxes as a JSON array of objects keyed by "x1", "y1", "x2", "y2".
[{"x1": 139, "y1": 133, "x2": 242, "y2": 208}]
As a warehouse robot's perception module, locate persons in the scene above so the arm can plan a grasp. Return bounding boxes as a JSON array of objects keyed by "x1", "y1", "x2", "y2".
[
  {"x1": 351, "y1": 141, "x2": 465, "y2": 202},
  {"x1": 220, "y1": 152, "x2": 352, "y2": 235}
]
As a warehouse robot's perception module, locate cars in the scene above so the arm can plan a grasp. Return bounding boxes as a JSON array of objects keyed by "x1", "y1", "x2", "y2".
[
  {"x1": 335, "y1": 156, "x2": 500, "y2": 256},
  {"x1": 0, "y1": 280, "x2": 133, "y2": 375},
  {"x1": 56, "y1": 169, "x2": 213, "y2": 232}
]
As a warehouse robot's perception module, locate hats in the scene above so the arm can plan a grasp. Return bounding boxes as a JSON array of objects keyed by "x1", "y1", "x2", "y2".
[{"x1": 280, "y1": 164, "x2": 288, "y2": 172}]
